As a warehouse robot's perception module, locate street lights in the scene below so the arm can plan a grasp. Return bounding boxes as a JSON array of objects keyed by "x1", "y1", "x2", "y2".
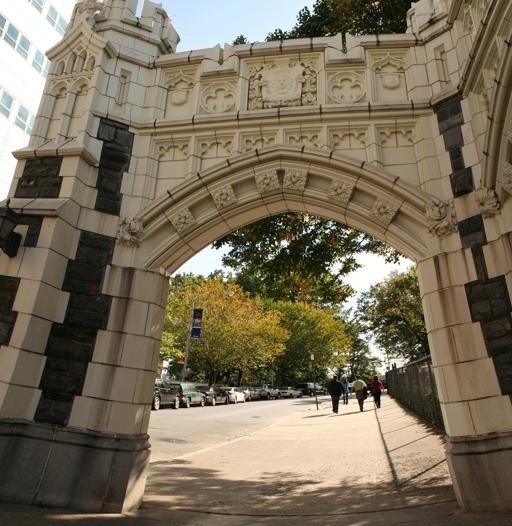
[{"x1": 310, "y1": 353, "x2": 319, "y2": 410}]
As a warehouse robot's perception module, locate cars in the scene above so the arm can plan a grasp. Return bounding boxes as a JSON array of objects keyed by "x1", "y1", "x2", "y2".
[{"x1": 152, "y1": 378, "x2": 328, "y2": 412}]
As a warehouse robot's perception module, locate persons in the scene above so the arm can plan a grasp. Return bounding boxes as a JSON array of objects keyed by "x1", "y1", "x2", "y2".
[
  {"x1": 327, "y1": 375, "x2": 346, "y2": 415},
  {"x1": 341, "y1": 379, "x2": 350, "y2": 405},
  {"x1": 370, "y1": 375, "x2": 384, "y2": 409},
  {"x1": 350, "y1": 375, "x2": 368, "y2": 412}
]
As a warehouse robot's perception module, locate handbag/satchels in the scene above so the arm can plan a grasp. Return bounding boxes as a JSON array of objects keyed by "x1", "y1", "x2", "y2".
[{"x1": 371, "y1": 384, "x2": 377, "y2": 393}]
